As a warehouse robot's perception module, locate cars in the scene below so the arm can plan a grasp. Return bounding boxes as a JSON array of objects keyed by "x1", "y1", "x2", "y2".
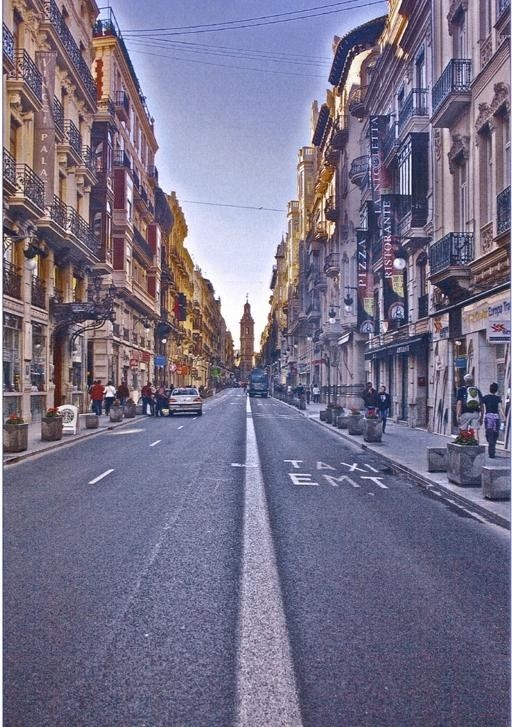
[{"x1": 169, "y1": 388, "x2": 202, "y2": 416}]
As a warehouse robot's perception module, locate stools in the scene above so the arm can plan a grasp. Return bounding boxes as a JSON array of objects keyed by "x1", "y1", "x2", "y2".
[
  {"x1": 337, "y1": 416, "x2": 349, "y2": 429},
  {"x1": 136, "y1": 404, "x2": 144, "y2": 415},
  {"x1": 482, "y1": 466, "x2": 511, "y2": 499},
  {"x1": 427, "y1": 447, "x2": 449, "y2": 472},
  {"x1": 86, "y1": 415, "x2": 98, "y2": 429}
]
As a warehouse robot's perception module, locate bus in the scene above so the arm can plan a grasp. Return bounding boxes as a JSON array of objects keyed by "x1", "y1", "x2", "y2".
[{"x1": 248, "y1": 369, "x2": 268, "y2": 397}]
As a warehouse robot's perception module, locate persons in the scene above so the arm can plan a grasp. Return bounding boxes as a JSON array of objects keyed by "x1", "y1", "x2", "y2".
[
  {"x1": 361, "y1": 381, "x2": 378, "y2": 417},
  {"x1": 376, "y1": 385, "x2": 392, "y2": 433},
  {"x1": 457, "y1": 373, "x2": 484, "y2": 437},
  {"x1": 81, "y1": 378, "x2": 171, "y2": 418},
  {"x1": 312, "y1": 384, "x2": 320, "y2": 402},
  {"x1": 274, "y1": 382, "x2": 287, "y2": 394},
  {"x1": 295, "y1": 381, "x2": 304, "y2": 398},
  {"x1": 478, "y1": 381, "x2": 506, "y2": 458},
  {"x1": 286, "y1": 382, "x2": 294, "y2": 396}
]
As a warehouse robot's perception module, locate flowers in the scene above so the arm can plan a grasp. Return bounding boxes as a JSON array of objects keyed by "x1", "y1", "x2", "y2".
[
  {"x1": 453, "y1": 427, "x2": 479, "y2": 445},
  {"x1": 46, "y1": 405, "x2": 60, "y2": 419},
  {"x1": 113, "y1": 399, "x2": 121, "y2": 405},
  {"x1": 6, "y1": 413, "x2": 24, "y2": 425},
  {"x1": 126, "y1": 398, "x2": 134, "y2": 405},
  {"x1": 350, "y1": 406, "x2": 360, "y2": 415},
  {"x1": 365, "y1": 407, "x2": 377, "y2": 419}
]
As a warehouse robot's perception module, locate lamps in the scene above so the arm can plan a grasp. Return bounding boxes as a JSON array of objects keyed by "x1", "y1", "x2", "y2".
[
  {"x1": 33, "y1": 327, "x2": 41, "y2": 350},
  {"x1": 133, "y1": 315, "x2": 219, "y2": 367},
  {"x1": 91, "y1": 272, "x2": 103, "y2": 306},
  {"x1": 454, "y1": 338, "x2": 465, "y2": 346},
  {"x1": 328, "y1": 305, "x2": 340, "y2": 326},
  {"x1": 2, "y1": 235, "x2": 40, "y2": 271},
  {"x1": 345, "y1": 287, "x2": 361, "y2": 312},
  {"x1": 108, "y1": 283, "x2": 117, "y2": 311},
  {"x1": 390, "y1": 236, "x2": 432, "y2": 270},
  {"x1": 266, "y1": 327, "x2": 319, "y2": 367}
]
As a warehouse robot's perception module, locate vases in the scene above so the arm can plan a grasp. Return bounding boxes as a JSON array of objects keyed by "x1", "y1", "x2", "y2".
[
  {"x1": 109, "y1": 405, "x2": 124, "y2": 422},
  {"x1": 363, "y1": 418, "x2": 384, "y2": 442},
  {"x1": 42, "y1": 417, "x2": 63, "y2": 441},
  {"x1": 447, "y1": 443, "x2": 486, "y2": 487},
  {"x1": 5, "y1": 425, "x2": 28, "y2": 453},
  {"x1": 348, "y1": 415, "x2": 364, "y2": 435},
  {"x1": 124, "y1": 404, "x2": 137, "y2": 418}
]
